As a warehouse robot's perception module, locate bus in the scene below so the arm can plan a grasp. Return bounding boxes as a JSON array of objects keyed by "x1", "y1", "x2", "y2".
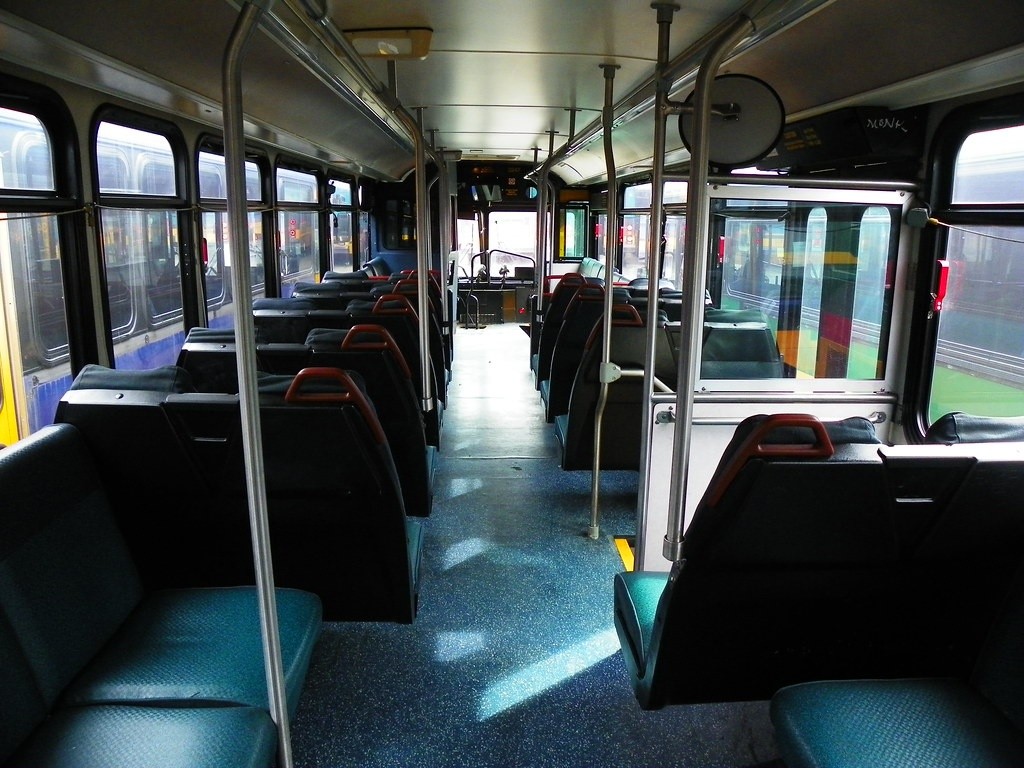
[
  {"x1": 1, "y1": 105, "x2": 364, "y2": 448},
  {"x1": 595, "y1": 142, "x2": 1024, "y2": 446}
]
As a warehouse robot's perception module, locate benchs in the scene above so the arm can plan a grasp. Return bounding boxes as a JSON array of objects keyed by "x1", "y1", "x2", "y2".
[
  {"x1": 528, "y1": 257, "x2": 1024, "y2": 768},
  {"x1": 0, "y1": 257, "x2": 450, "y2": 767}
]
